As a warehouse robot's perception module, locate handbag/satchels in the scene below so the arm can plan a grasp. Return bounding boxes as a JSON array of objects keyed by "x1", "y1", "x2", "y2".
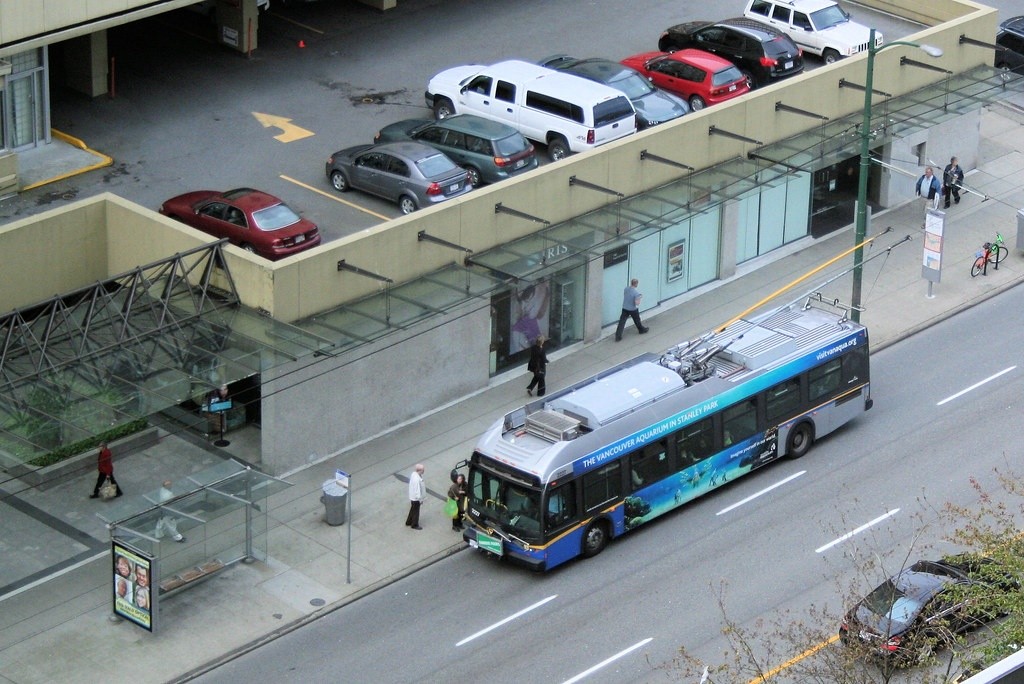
[
  {"x1": 443, "y1": 488, "x2": 458, "y2": 519},
  {"x1": 942, "y1": 187, "x2": 946, "y2": 195},
  {"x1": 98, "y1": 478, "x2": 117, "y2": 500},
  {"x1": 535, "y1": 358, "x2": 546, "y2": 375}
]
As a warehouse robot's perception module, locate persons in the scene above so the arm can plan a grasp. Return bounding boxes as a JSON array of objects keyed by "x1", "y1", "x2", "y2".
[
  {"x1": 916, "y1": 167, "x2": 941, "y2": 229},
  {"x1": 405, "y1": 464, "x2": 428, "y2": 530},
  {"x1": 116, "y1": 577, "x2": 127, "y2": 599},
  {"x1": 156, "y1": 478, "x2": 187, "y2": 543},
  {"x1": 134, "y1": 563, "x2": 149, "y2": 589},
  {"x1": 134, "y1": 585, "x2": 149, "y2": 614},
  {"x1": 448, "y1": 474, "x2": 467, "y2": 531},
  {"x1": 511, "y1": 276, "x2": 548, "y2": 352},
  {"x1": 615, "y1": 279, "x2": 649, "y2": 341},
  {"x1": 943, "y1": 156, "x2": 964, "y2": 209},
  {"x1": 537, "y1": 493, "x2": 571, "y2": 526},
  {"x1": 628, "y1": 429, "x2": 732, "y2": 491},
  {"x1": 116, "y1": 555, "x2": 131, "y2": 579},
  {"x1": 88, "y1": 440, "x2": 123, "y2": 498},
  {"x1": 525, "y1": 334, "x2": 549, "y2": 397}
]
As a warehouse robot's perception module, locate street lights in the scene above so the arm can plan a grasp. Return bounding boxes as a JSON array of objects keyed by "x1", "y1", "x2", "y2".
[{"x1": 851, "y1": 26, "x2": 944, "y2": 325}]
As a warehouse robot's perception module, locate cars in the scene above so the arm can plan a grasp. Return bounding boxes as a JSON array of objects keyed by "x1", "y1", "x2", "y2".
[
  {"x1": 158, "y1": 187, "x2": 321, "y2": 262},
  {"x1": 537, "y1": 54, "x2": 691, "y2": 132},
  {"x1": 839, "y1": 552, "x2": 1022, "y2": 670},
  {"x1": 995, "y1": 15, "x2": 1024, "y2": 83},
  {"x1": 619, "y1": 48, "x2": 753, "y2": 114},
  {"x1": 325, "y1": 140, "x2": 473, "y2": 214}
]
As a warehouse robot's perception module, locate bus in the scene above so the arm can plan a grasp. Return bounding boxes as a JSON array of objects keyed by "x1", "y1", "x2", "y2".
[{"x1": 450, "y1": 224, "x2": 914, "y2": 573}]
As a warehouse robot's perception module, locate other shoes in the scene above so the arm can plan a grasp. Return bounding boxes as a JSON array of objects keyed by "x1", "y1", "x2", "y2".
[
  {"x1": 639, "y1": 328, "x2": 649, "y2": 334},
  {"x1": 406, "y1": 522, "x2": 412, "y2": 525},
  {"x1": 89, "y1": 493, "x2": 99, "y2": 498},
  {"x1": 527, "y1": 389, "x2": 532, "y2": 396},
  {"x1": 176, "y1": 538, "x2": 187, "y2": 542},
  {"x1": 615, "y1": 337, "x2": 622, "y2": 341},
  {"x1": 921, "y1": 224, "x2": 925, "y2": 229},
  {"x1": 954, "y1": 196, "x2": 960, "y2": 204},
  {"x1": 459, "y1": 525, "x2": 465, "y2": 529},
  {"x1": 412, "y1": 525, "x2": 422, "y2": 530},
  {"x1": 944, "y1": 205, "x2": 950, "y2": 209},
  {"x1": 114, "y1": 492, "x2": 122, "y2": 497},
  {"x1": 452, "y1": 526, "x2": 460, "y2": 532}
]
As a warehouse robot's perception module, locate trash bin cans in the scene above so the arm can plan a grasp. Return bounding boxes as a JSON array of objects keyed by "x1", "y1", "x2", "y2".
[{"x1": 320, "y1": 478, "x2": 347, "y2": 526}]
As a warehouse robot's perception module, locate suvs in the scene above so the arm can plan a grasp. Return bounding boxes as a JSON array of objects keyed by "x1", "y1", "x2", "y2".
[
  {"x1": 374, "y1": 113, "x2": 539, "y2": 188},
  {"x1": 425, "y1": 60, "x2": 637, "y2": 162},
  {"x1": 658, "y1": 15, "x2": 805, "y2": 91},
  {"x1": 743, "y1": 0, "x2": 884, "y2": 66}
]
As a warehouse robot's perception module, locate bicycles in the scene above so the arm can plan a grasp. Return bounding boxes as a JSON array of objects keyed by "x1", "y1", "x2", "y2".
[{"x1": 971, "y1": 231, "x2": 1009, "y2": 278}]
